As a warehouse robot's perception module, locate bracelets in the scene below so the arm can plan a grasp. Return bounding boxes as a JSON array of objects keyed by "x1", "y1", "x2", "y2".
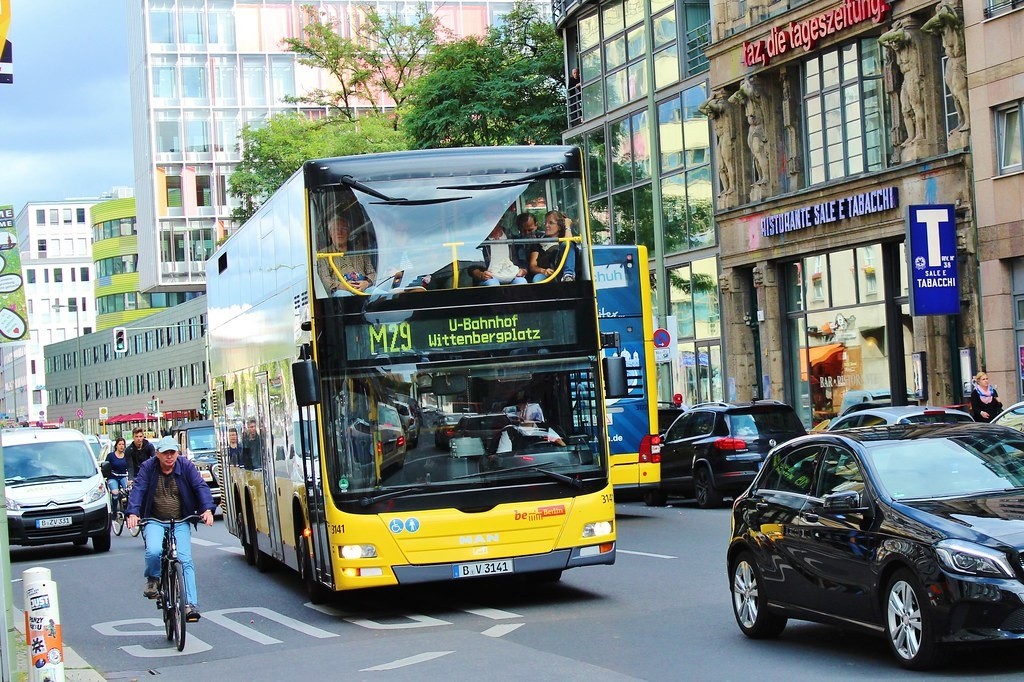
[
  {"x1": 207, "y1": 509, "x2": 212, "y2": 513},
  {"x1": 565, "y1": 227, "x2": 572, "y2": 231}
]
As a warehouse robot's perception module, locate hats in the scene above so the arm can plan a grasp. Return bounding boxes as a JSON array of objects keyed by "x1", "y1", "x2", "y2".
[{"x1": 155, "y1": 436, "x2": 181, "y2": 453}]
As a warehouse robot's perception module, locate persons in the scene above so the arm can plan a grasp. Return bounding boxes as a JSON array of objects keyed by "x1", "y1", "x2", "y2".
[
  {"x1": 877, "y1": 20, "x2": 925, "y2": 146},
  {"x1": 229, "y1": 418, "x2": 270, "y2": 472},
  {"x1": 971, "y1": 371, "x2": 1003, "y2": 424},
  {"x1": 568, "y1": 68, "x2": 582, "y2": 127},
  {"x1": 495, "y1": 396, "x2": 566, "y2": 453},
  {"x1": 727, "y1": 79, "x2": 770, "y2": 187},
  {"x1": 124, "y1": 427, "x2": 156, "y2": 486},
  {"x1": 124, "y1": 436, "x2": 217, "y2": 623},
  {"x1": 919, "y1": 6, "x2": 971, "y2": 135},
  {"x1": 105, "y1": 437, "x2": 128, "y2": 520},
  {"x1": 697, "y1": 91, "x2": 737, "y2": 198},
  {"x1": 317, "y1": 199, "x2": 576, "y2": 297}
]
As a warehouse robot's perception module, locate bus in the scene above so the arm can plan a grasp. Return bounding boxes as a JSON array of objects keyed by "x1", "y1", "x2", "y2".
[
  {"x1": 577, "y1": 246, "x2": 660, "y2": 491},
  {"x1": 203, "y1": 145, "x2": 630, "y2": 605}
]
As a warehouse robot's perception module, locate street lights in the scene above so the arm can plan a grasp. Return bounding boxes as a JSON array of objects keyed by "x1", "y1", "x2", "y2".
[{"x1": 52, "y1": 304, "x2": 84, "y2": 433}]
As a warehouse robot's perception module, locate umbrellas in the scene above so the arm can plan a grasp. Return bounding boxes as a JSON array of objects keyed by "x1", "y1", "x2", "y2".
[{"x1": 98, "y1": 412, "x2": 158, "y2": 431}]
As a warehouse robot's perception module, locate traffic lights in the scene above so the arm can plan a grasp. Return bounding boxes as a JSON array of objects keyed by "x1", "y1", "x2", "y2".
[
  {"x1": 115, "y1": 327, "x2": 128, "y2": 352},
  {"x1": 147, "y1": 400, "x2": 154, "y2": 411},
  {"x1": 201, "y1": 398, "x2": 207, "y2": 410}
]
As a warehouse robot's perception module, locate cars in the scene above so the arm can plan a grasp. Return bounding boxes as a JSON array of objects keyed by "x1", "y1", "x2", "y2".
[
  {"x1": 822, "y1": 389, "x2": 1024, "y2": 432},
  {"x1": 170, "y1": 422, "x2": 222, "y2": 520},
  {"x1": 82, "y1": 434, "x2": 161, "y2": 480},
  {"x1": 726, "y1": 422, "x2": 1024, "y2": 672}
]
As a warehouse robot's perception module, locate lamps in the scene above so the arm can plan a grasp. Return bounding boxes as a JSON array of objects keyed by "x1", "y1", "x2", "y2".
[
  {"x1": 911, "y1": 351, "x2": 927, "y2": 400},
  {"x1": 958, "y1": 345, "x2": 978, "y2": 398}
]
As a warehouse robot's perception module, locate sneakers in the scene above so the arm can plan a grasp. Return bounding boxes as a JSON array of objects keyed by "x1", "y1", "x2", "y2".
[
  {"x1": 143, "y1": 577, "x2": 160, "y2": 595},
  {"x1": 186, "y1": 606, "x2": 201, "y2": 622}
]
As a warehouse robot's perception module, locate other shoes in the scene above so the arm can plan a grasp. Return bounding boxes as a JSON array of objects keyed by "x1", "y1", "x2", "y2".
[{"x1": 561, "y1": 273, "x2": 574, "y2": 282}]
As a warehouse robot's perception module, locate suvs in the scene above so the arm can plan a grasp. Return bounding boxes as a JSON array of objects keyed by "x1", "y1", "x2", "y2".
[
  {"x1": 641, "y1": 399, "x2": 808, "y2": 509},
  {"x1": 656, "y1": 401, "x2": 688, "y2": 441}
]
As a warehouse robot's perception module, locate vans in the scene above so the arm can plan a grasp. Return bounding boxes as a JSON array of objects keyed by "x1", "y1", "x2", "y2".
[{"x1": 1, "y1": 426, "x2": 113, "y2": 556}]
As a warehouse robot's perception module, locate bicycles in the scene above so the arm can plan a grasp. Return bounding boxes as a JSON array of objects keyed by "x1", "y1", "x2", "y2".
[
  {"x1": 107, "y1": 470, "x2": 140, "y2": 538},
  {"x1": 130, "y1": 515, "x2": 213, "y2": 652}
]
